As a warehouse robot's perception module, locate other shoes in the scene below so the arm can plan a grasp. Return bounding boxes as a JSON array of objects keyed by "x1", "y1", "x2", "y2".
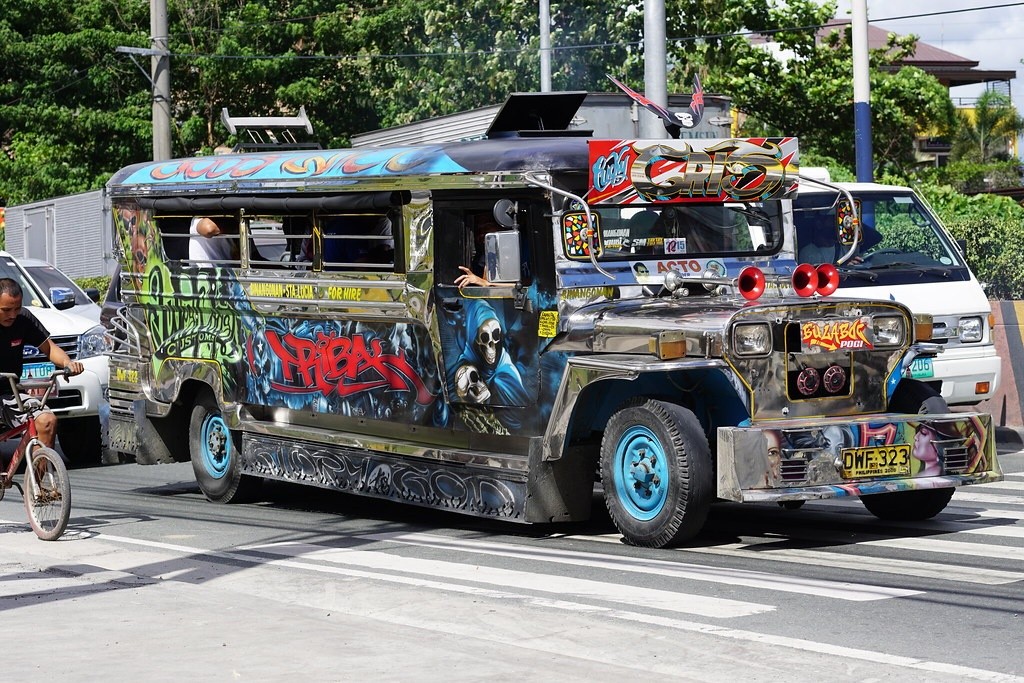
[{"x1": 37, "y1": 489, "x2": 63, "y2": 505}]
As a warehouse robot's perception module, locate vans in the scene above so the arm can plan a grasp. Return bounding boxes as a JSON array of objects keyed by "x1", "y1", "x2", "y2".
[{"x1": 625, "y1": 165, "x2": 1001, "y2": 404}]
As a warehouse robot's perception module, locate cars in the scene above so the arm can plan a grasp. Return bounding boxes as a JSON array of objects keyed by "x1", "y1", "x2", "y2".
[{"x1": 16, "y1": 257, "x2": 102, "y2": 322}]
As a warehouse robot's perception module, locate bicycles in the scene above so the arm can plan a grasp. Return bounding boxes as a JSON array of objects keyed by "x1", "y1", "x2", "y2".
[{"x1": 0, "y1": 368, "x2": 83, "y2": 541}]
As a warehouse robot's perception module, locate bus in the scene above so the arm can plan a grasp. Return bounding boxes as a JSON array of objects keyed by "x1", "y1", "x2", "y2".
[{"x1": 98, "y1": 74, "x2": 1005, "y2": 550}]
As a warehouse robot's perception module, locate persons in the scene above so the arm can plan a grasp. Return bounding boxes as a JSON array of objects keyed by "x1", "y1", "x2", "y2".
[
  {"x1": 0, "y1": 278, "x2": 83, "y2": 498},
  {"x1": 635, "y1": 262, "x2": 650, "y2": 277},
  {"x1": 187, "y1": 214, "x2": 237, "y2": 268},
  {"x1": 353, "y1": 207, "x2": 395, "y2": 271},
  {"x1": 454, "y1": 211, "x2": 533, "y2": 288},
  {"x1": 764, "y1": 429, "x2": 781, "y2": 486}
]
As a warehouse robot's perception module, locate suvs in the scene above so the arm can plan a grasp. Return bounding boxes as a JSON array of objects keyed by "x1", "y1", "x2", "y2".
[{"x1": 0, "y1": 250, "x2": 111, "y2": 465}]
